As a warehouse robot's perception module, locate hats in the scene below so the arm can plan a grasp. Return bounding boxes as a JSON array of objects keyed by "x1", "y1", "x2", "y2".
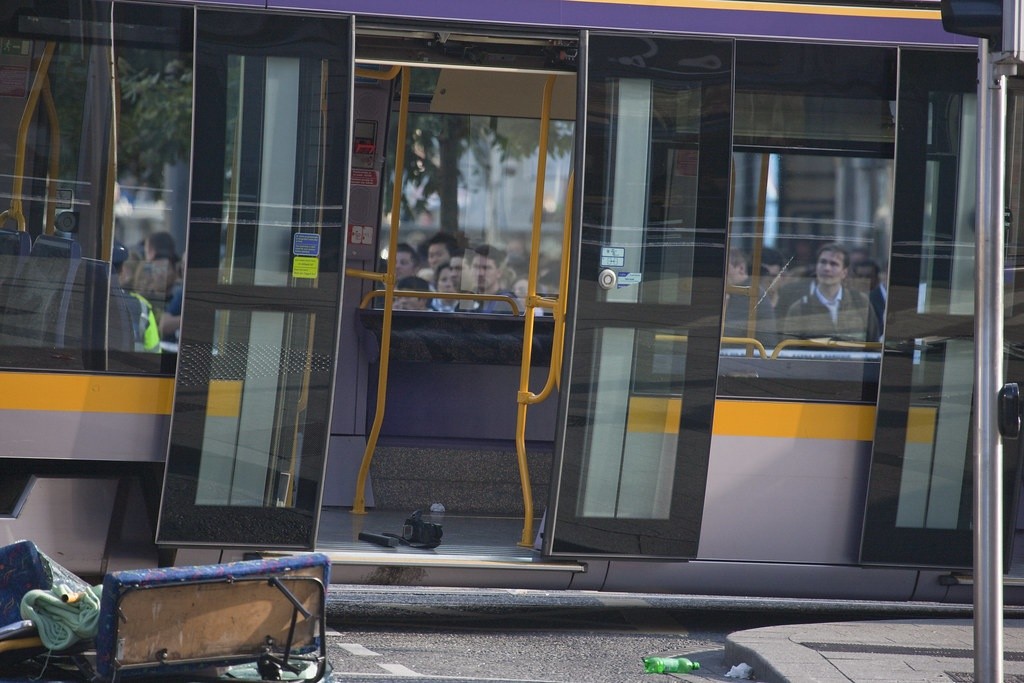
[{"x1": 113, "y1": 244, "x2": 128, "y2": 264}]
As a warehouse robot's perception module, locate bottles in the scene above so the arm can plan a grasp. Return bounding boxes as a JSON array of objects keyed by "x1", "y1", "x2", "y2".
[{"x1": 643, "y1": 657, "x2": 701, "y2": 673}]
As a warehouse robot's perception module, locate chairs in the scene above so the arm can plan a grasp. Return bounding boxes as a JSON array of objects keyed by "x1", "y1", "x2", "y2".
[{"x1": 0, "y1": 229, "x2": 136, "y2": 352}]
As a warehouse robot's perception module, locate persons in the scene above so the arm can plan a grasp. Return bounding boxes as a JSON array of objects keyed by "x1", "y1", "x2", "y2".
[
  {"x1": 728, "y1": 242, "x2": 884, "y2": 344},
  {"x1": 380, "y1": 236, "x2": 558, "y2": 316},
  {"x1": 471, "y1": 244, "x2": 523, "y2": 312},
  {"x1": 113, "y1": 220, "x2": 186, "y2": 350}
]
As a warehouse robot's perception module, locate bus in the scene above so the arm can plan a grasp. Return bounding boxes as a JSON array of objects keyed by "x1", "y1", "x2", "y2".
[{"x1": 2, "y1": 2, "x2": 1021, "y2": 614}]
